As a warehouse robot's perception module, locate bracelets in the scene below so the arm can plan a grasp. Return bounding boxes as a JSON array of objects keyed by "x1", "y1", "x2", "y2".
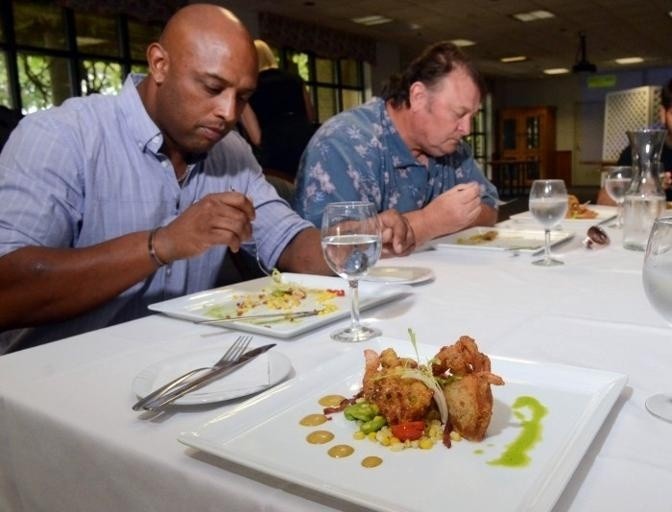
[{"x1": 148, "y1": 226, "x2": 171, "y2": 269}]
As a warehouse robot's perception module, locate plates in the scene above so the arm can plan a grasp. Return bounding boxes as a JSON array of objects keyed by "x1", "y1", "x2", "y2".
[
  {"x1": 133, "y1": 346, "x2": 293, "y2": 409},
  {"x1": 146, "y1": 271, "x2": 416, "y2": 341},
  {"x1": 429, "y1": 226, "x2": 574, "y2": 256},
  {"x1": 177, "y1": 337, "x2": 628, "y2": 512},
  {"x1": 360, "y1": 261, "x2": 437, "y2": 286},
  {"x1": 510, "y1": 201, "x2": 624, "y2": 228}
]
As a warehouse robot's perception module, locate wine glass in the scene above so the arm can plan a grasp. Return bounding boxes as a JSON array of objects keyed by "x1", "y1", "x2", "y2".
[
  {"x1": 527, "y1": 179, "x2": 568, "y2": 267},
  {"x1": 640, "y1": 215, "x2": 672, "y2": 425},
  {"x1": 321, "y1": 201, "x2": 386, "y2": 342},
  {"x1": 605, "y1": 167, "x2": 638, "y2": 230}
]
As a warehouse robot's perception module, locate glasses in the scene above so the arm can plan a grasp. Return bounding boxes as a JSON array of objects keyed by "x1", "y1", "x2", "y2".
[{"x1": 583, "y1": 226, "x2": 610, "y2": 250}]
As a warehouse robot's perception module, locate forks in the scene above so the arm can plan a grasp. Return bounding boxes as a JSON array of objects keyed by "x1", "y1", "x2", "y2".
[
  {"x1": 228, "y1": 185, "x2": 288, "y2": 290},
  {"x1": 456, "y1": 187, "x2": 522, "y2": 206},
  {"x1": 131, "y1": 342, "x2": 250, "y2": 412}
]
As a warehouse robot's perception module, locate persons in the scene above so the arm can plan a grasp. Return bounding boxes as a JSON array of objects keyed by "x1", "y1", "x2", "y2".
[
  {"x1": 292, "y1": 42, "x2": 499, "y2": 264},
  {"x1": 241, "y1": 40, "x2": 315, "y2": 194},
  {"x1": 0, "y1": 1, "x2": 416, "y2": 356},
  {"x1": 596, "y1": 78, "x2": 672, "y2": 206}
]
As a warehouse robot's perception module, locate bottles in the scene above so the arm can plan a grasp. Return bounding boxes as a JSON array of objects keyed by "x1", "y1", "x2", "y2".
[{"x1": 618, "y1": 125, "x2": 668, "y2": 253}]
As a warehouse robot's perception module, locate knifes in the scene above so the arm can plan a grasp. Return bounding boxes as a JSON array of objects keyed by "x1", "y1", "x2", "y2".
[
  {"x1": 146, "y1": 342, "x2": 279, "y2": 414},
  {"x1": 195, "y1": 310, "x2": 326, "y2": 333}
]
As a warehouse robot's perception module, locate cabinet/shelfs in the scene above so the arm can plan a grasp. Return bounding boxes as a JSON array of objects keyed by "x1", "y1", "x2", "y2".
[{"x1": 499, "y1": 105, "x2": 558, "y2": 188}]
